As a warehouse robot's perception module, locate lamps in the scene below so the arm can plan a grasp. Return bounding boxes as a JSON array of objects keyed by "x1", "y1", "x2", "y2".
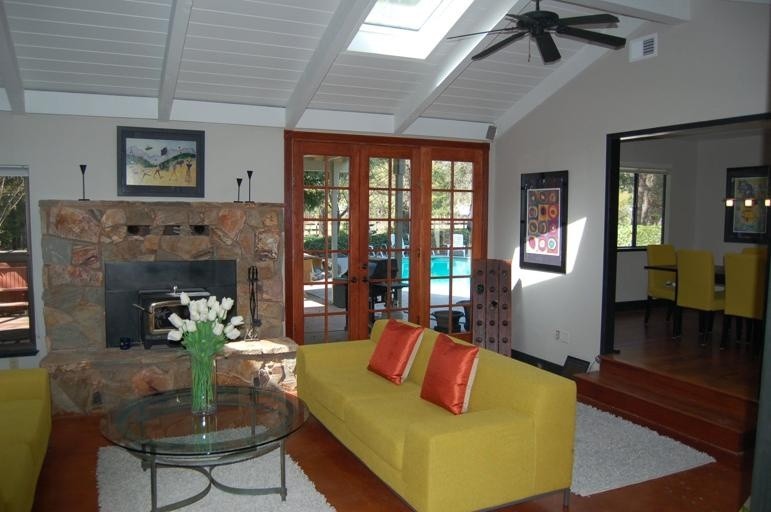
[
  {"x1": 723, "y1": 199, "x2": 735, "y2": 207},
  {"x1": 527, "y1": 21, "x2": 546, "y2": 64},
  {"x1": 764, "y1": 199, "x2": 770, "y2": 207},
  {"x1": 743, "y1": 198, "x2": 755, "y2": 207}
]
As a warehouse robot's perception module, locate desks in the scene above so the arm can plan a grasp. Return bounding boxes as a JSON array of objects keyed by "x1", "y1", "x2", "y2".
[
  {"x1": 344, "y1": 281, "x2": 409, "y2": 331},
  {"x1": 644, "y1": 265, "x2": 726, "y2": 286}
]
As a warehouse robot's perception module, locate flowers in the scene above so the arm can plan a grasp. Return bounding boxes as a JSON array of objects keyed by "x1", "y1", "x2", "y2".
[{"x1": 166, "y1": 290, "x2": 242, "y2": 415}]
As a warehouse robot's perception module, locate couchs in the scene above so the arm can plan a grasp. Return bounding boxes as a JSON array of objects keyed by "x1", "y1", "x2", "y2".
[
  {"x1": 332, "y1": 258, "x2": 397, "y2": 308},
  {"x1": 299, "y1": 321, "x2": 578, "y2": 511},
  {"x1": 0, "y1": 368, "x2": 55, "y2": 512}
]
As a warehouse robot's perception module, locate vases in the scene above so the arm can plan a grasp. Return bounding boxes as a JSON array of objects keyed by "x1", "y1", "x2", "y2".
[
  {"x1": 456, "y1": 300, "x2": 471, "y2": 328},
  {"x1": 432, "y1": 310, "x2": 464, "y2": 328},
  {"x1": 187, "y1": 351, "x2": 218, "y2": 415}
]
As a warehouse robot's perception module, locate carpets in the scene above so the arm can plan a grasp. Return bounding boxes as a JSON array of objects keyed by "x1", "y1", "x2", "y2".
[
  {"x1": 568, "y1": 401, "x2": 716, "y2": 498},
  {"x1": 95, "y1": 424, "x2": 336, "y2": 512}
]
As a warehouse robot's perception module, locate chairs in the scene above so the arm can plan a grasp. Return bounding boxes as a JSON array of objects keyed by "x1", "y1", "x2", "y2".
[
  {"x1": 644, "y1": 243, "x2": 678, "y2": 337},
  {"x1": 721, "y1": 251, "x2": 766, "y2": 353},
  {"x1": 740, "y1": 246, "x2": 771, "y2": 345},
  {"x1": 672, "y1": 247, "x2": 727, "y2": 346}
]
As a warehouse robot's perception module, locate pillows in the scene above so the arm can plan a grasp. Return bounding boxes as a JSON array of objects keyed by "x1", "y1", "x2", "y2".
[
  {"x1": 419, "y1": 332, "x2": 480, "y2": 415},
  {"x1": 367, "y1": 317, "x2": 429, "y2": 387}
]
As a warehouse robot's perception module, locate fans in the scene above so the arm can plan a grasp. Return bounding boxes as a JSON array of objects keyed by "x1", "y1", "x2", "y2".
[{"x1": 446, "y1": 0, "x2": 627, "y2": 64}]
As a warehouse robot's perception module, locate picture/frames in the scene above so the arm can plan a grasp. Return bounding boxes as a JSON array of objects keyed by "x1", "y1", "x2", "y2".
[
  {"x1": 116, "y1": 125, "x2": 205, "y2": 198},
  {"x1": 519, "y1": 169, "x2": 568, "y2": 275},
  {"x1": 723, "y1": 164, "x2": 770, "y2": 245}
]
